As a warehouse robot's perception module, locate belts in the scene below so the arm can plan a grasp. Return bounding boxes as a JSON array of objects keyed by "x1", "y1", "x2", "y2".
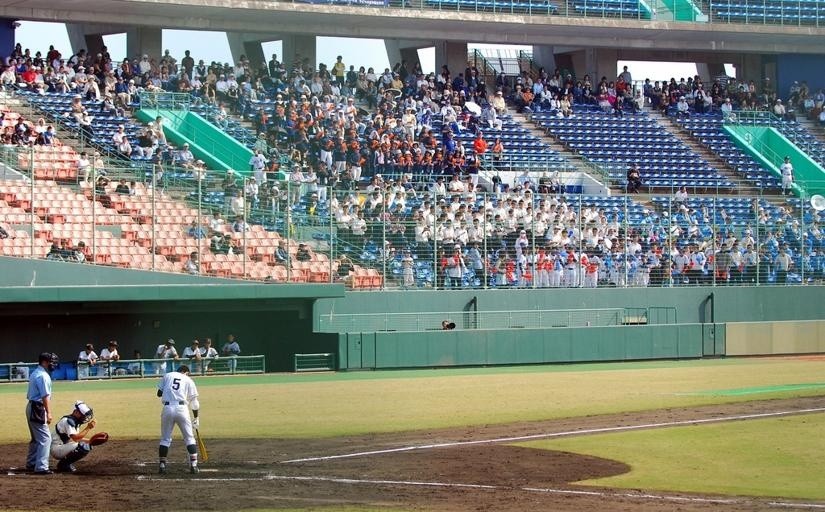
[{"x1": 165, "y1": 402, "x2": 184, "y2": 405}]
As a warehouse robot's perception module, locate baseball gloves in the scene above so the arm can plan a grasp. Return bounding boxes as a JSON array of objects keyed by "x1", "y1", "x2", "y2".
[{"x1": 89, "y1": 432, "x2": 108, "y2": 445}]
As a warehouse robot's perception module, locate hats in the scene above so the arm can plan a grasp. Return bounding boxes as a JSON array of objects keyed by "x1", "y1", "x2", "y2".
[{"x1": 86, "y1": 336, "x2": 212, "y2": 350}]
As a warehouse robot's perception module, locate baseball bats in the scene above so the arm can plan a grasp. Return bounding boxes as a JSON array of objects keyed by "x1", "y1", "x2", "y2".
[{"x1": 197, "y1": 429, "x2": 207, "y2": 462}]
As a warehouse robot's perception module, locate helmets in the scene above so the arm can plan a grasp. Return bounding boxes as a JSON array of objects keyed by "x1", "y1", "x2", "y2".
[
  {"x1": 74, "y1": 400, "x2": 93, "y2": 426},
  {"x1": 39, "y1": 352, "x2": 60, "y2": 372}
]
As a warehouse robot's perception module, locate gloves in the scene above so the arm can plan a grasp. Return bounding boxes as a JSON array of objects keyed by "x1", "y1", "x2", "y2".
[{"x1": 192, "y1": 417, "x2": 199, "y2": 428}]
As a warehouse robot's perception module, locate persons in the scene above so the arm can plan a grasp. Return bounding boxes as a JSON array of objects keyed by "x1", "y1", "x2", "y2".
[
  {"x1": 25, "y1": 353, "x2": 59, "y2": 474},
  {"x1": 0, "y1": 44, "x2": 824, "y2": 291},
  {"x1": 156, "y1": 365, "x2": 200, "y2": 474},
  {"x1": 76, "y1": 333, "x2": 241, "y2": 379},
  {"x1": 50, "y1": 400, "x2": 96, "y2": 472},
  {"x1": 442, "y1": 320, "x2": 450, "y2": 329}
]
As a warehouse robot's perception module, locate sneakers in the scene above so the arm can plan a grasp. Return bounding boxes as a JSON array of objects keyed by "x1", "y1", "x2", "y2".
[
  {"x1": 35, "y1": 461, "x2": 76, "y2": 474},
  {"x1": 159, "y1": 464, "x2": 167, "y2": 474},
  {"x1": 191, "y1": 464, "x2": 199, "y2": 472}
]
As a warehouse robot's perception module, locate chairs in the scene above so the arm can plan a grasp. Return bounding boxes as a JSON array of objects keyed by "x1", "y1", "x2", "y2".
[
  {"x1": 0, "y1": 360, "x2": 209, "y2": 380},
  {"x1": 518, "y1": 95, "x2": 825, "y2": 190},
  {"x1": 277, "y1": 0, "x2": 825, "y2": 27},
  {"x1": 0, "y1": 80, "x2": 278, "y2": 179},
  {"x1": 223, "y1": 83, "x2": 580, "y2": 177},
  {"x1": 1, "y1": 181, "x2": 383, "y2": 290},
  {"x1": 382, "y1": 176, "x2": 825, "y2": 288}
]
